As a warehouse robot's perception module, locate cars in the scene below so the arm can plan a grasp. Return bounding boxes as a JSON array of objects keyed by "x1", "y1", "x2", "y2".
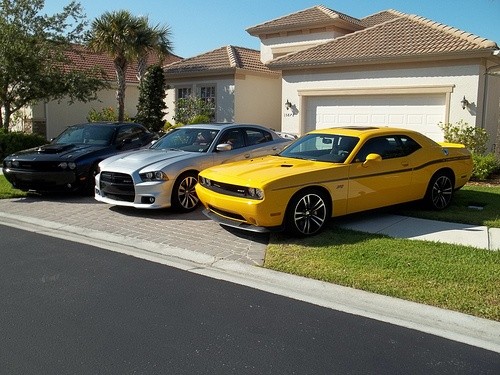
[
  {"x1": 195, "y1": 125, "x2": 474, "y2": 238},
  {"x1": 3, "y1": 120, "x2": 162, "y2": 199},
  {"x1": 93, "y1": 121, "x2": 299, "y2": 213}
]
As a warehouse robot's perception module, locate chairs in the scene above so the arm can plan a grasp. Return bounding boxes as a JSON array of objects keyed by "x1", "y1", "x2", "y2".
[
  {"x1": 330, "y1": 137, "x2": 355, "y2": 154},
  {"x1": 190, "y1": 132, "x2": 243, "y2": 147}
]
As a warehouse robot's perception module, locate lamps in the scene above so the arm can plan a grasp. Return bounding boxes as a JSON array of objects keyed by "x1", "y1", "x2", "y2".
[
  {"x1": 285, "y1": 100, "x2": 292, "y2": 111},
  {"x1": 460, "y1": 96, "x2": 469, "y2": 110}
]
